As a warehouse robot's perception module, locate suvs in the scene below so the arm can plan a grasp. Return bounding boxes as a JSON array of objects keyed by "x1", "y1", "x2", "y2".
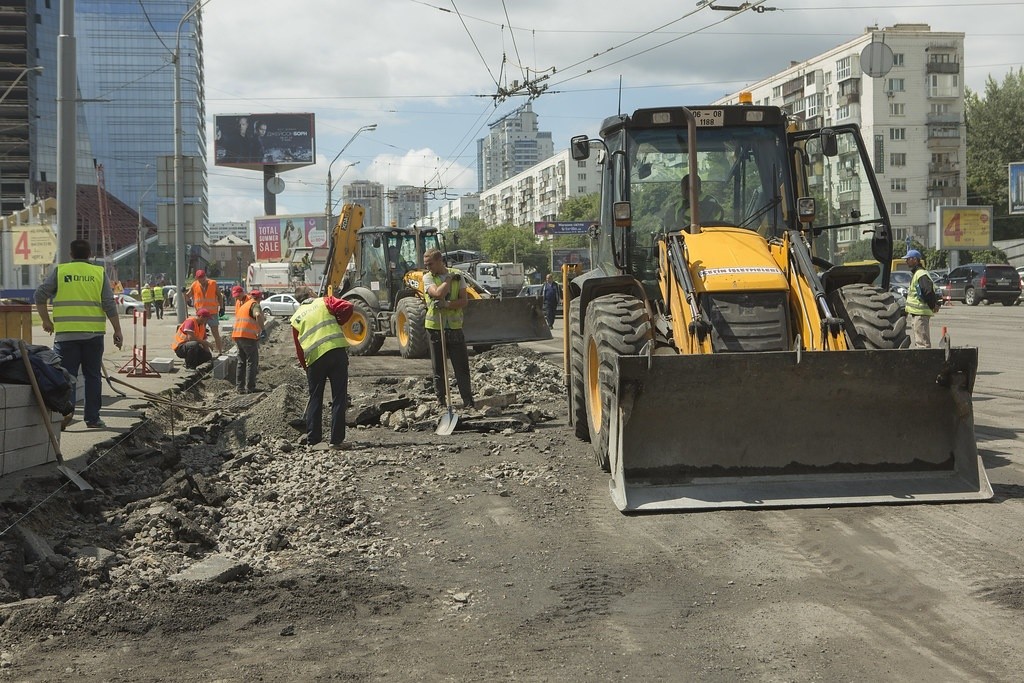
[{"x1": 933, "y1": 264, "x2": 1022, "y2": 306}]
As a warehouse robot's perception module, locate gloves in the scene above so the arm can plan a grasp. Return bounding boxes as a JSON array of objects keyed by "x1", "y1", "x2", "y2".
[
  {"x1": 432, "y1": 299, "x2": 448, "y2": 309},
  {"x1": 219, "y1": 309, "x2": 225, "y2": 317},
  {"x1": 447, "y1": 272, "x2": 456, "y2": 281},
  {"x1": 261, "y1": 330, "x2": 267, "y2": 341}
]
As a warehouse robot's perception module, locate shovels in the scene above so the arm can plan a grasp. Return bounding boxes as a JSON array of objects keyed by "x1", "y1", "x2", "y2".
[
  {"x1": 288, "y1": 396, "x2": 311, "y2": 425},
  {"x1": 434, "y1": 308, "x2": 458, "y2": 435}
]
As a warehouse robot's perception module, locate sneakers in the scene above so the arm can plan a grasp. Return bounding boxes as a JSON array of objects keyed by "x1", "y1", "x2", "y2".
[
  {"x1": 185, "y1": 364, "x2": 197, "y2": 370},
  {"x1": 61, "y1": 414, "x2": 73, "y2": 431},
  {"x1": 86, "y1": 419, "x2": 105, "y2": 428}
]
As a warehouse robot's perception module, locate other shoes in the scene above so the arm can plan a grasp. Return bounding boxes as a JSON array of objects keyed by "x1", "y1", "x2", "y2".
[
  {"x1": 464, "y1": 404, "x2": 477, "y2": 414},
  {"x1": 437, "y1": 402, "x2": 446, "y2": 408}
]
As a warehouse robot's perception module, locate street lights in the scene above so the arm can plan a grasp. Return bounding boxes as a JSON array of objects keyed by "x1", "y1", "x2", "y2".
[{"x1": 325, "y1": 124, "x2": 379, "y2": 246}]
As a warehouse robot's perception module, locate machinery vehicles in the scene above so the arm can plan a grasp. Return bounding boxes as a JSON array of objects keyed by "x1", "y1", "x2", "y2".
[
  {"x1": 571, "y1": 93, "x2": 997, "y2": 514},
  {"x1": 320, "y1": 203, "x2": 554, "y2": 358},
  {"x1": 246, "y1": 247, "x2": 328, "y2": 294}
]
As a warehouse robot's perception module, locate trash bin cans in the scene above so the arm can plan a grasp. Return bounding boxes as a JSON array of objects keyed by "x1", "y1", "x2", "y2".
[
  {"x1": 101, "y1": 361, "x2": 127, "y2": 397},
  {"x1": 0, "y1": 298, "x2": 32, "y2": 345},
  {"x1": 18, "y1": 340, "x2": 94, "y2": 491}
]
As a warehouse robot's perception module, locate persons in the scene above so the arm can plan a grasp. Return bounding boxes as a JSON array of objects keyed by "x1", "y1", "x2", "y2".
[
  {"x1": 301, "y1": 253, "x2": 310, "y2": 269},
  {"x1": 422, "y1": 249, "x2": 484, "y2": 418},
  {"x1": 288, "y1": 286, "x2": 355, "y2": 449},
  {"x1": 538, "y1": 274, "x2": 563, "y2": 329},
  {"x1": 283, "y1": 219, "x2": 302, "y2": 247},
  {"x1": 34, "y1": 240, "x2": 123, "y2": 430},
  {"x1": 374, "y1": 244, "x2": 412, "y2": 283},
  {"x1": 232, "y1": 289, "x2": 266, "y2": 395},
  {"x1": 139, "y1": 281, "x2": 284, "y2": 320},
  {"x1": 185, "y1": 269, "x2": 226, "y2": 356},
  {"x1": 645, "y1": 173, "x2": 724, "y2": 270},
  {"x1": 901, "y1": 250, "x2": 942, "y2": 350},
  {"x1": 225, "y1": 116, "x2": 276, "y2": 163},
  {"x1": 172, "y1": 309, "x2": 214, "y2": 371}
]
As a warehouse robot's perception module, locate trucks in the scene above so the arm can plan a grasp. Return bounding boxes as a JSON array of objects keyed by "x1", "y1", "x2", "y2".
[
  {"x1": 451, "y1": 263, "x2": 502, "y2": 301},
  {"x1": 497, "y1": 263, "x2": 524, "y2": 297}
]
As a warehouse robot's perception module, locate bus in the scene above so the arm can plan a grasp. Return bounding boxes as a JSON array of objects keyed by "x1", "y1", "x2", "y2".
[{"x1": 843, "y1": 260, "x2": 925, "y2": 273}]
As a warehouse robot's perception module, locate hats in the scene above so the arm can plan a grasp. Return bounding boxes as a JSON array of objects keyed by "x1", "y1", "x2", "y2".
[
  {"x1": 144, "y1": 283, "x2": 149, "y2": 288},
  {"x1": 196, "y1": 270, "x2": 206, "y2": 279},
  {"x1": 250, "y1": 290, "x2": 261, "y2": 297},
  {"x1": 902, "y1": 250, "x2": 921, "y2": 259},
  {"x1": 232, "y1": 286, "x2": 244, "y2": 298},
  {"x1": 197, "y1": 308, "x2": 212, "y2": 320}
]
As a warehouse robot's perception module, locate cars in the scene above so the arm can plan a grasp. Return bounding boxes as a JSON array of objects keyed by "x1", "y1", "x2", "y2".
[
  {"x1": 1014, "y1": 267, "x2": 1024, "y2": 306},
  {"x1": 889, "y1": 270, "x2": 943, "y2": 313},
  {"x1": 259, "y1": 294, "x2": 303, "y2": 316},
  {"x1": 113, "y1": 294, "x2": 155, "y2": 315},
  {"x1": 516, "y1": 285, "x2": 544, "y2": 298},
  {"x1": 929, "y1": 271, "x2": 948, "y2": 280}
]
